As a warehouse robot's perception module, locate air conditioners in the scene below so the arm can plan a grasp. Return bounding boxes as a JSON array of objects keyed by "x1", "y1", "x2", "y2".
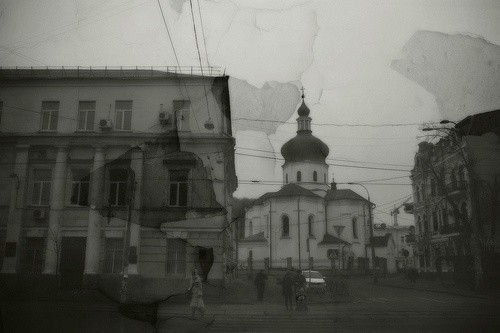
[
  {"x1": 31, "y1": 208, "x2": 46, "y2": 220},
  {"x1": 98, "y1": 118, "x2": 112, "y2": 128},
  {"x1": 157, "y1": 111, "x2": 169, "y2": 120}
]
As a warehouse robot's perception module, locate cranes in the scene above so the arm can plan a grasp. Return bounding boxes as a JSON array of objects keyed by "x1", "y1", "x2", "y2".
[{"x1": 391, "y1": 197, "x2": 412, "y2": 226}]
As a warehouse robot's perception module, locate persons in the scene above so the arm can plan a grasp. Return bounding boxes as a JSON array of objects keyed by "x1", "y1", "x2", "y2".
[
  {"x1": 295, "y1": 270, "x2": 308, "y2": 307},
  {"x1": 188, "y1": 268, "x2": 208, "y2": 321},
  {"x1": 226, "y1": 264, "x2": 239, "y2": 283},
  {"x1": 283, "y1": 272, "x2": 294, "y2": 312},
  {"x1": 254, "y1": 269, "x2": 268, "y2": 302}
]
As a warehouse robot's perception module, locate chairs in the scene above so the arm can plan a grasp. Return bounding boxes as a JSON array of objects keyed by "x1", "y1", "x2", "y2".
[{"x1": 295, "y1": 281, "x2": 307, "y2": 310}]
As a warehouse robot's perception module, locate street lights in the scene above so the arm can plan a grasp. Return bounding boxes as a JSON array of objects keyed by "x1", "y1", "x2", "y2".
[
  {"x1": 422, "y1": 127, "x2": 467, "y2": 167},
  {"x1": 347, "y1": 183, "x2": 378, "y2": 286},
  {"x1": 440, "y1": 120, "x2": 482, "y2": 234}
]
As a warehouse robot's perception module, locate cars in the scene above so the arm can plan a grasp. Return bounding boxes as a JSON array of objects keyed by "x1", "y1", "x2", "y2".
[{"x1": 300, "y1": 270, "x2": 327, "y2": 292}]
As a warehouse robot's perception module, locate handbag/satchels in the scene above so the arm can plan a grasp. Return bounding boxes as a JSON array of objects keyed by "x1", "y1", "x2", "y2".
[{"x1": 187, "y1": 287, "x2": 192, "y2": 295}]
